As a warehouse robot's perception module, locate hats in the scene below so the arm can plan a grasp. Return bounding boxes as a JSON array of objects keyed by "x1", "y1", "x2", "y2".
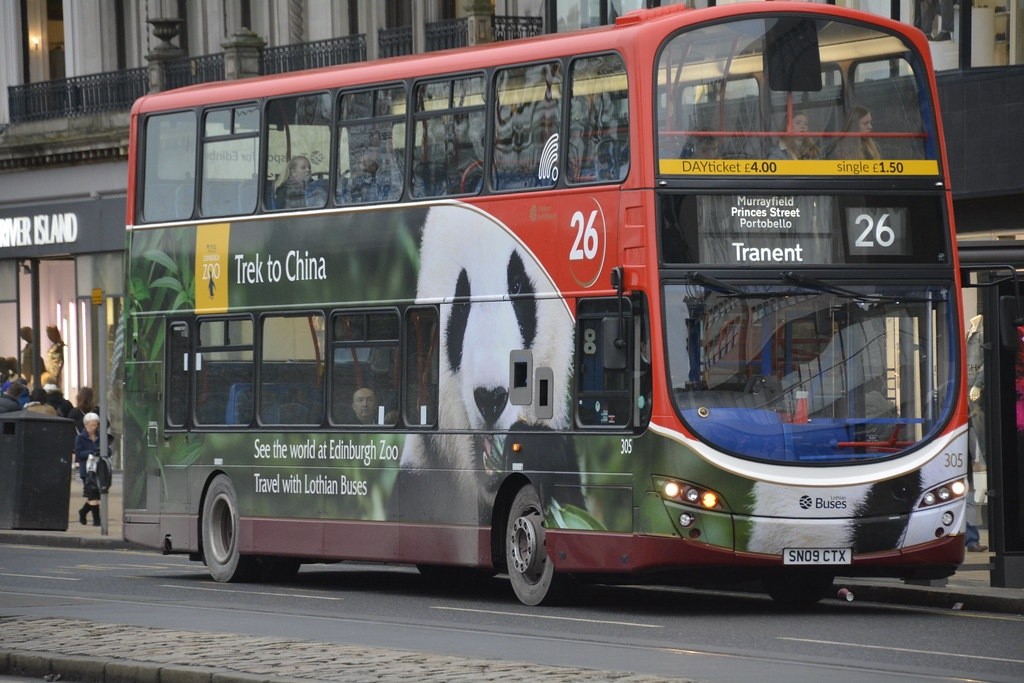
[{"x1": 43, "y1": 383, "x2": 57, "y2": 394}]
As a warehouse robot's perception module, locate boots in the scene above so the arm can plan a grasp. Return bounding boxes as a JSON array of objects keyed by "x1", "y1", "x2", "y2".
[
  {"x1": 92, "y1": 504, "x2": 102, "y2": 526},
  {"x1": 79, "y1": 502, "x2": 93, "y2": 526}
]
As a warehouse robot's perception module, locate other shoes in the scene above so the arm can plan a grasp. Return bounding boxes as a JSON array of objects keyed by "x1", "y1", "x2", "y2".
[{"x1": 968, "y1": 544, "x2": 988, "y2": 553}]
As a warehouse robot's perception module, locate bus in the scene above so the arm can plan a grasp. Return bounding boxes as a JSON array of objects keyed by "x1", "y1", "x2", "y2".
[{"x1": 119, "y1": 0, "x2": 1024, "y2": 607}]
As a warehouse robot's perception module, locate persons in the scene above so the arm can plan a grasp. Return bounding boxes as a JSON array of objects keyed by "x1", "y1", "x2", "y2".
[
  {"x1": 688, "y1": 129, "x2": 722, "y2": 160},
  {"x1": 0, "y1": 372, "x2": 74, "y2": 418},
  {"x1": 73, "y1": 412, "x2": 112, "y2": 526},
  {"x1": 21, "y1": 327, "x2": 35, "y2": 383},
  {"x1": 348, "y1": 388, "x2": 378, "y2": 424},
  {"x1": 638, "y1": 317, "x2": 687, "y2": 396},
  {"x1": 68, "y1": 386, "x2": 111, "y2": 453},
  {"x1": 827, "y1": 105, "x2": 884, "y2": 161},
  {"x1": 964, "y1": 451, "x2": 988, "y2": 553},
  {"x1": 45, "y1": 325, "x2": 65, "y2": 376},
  {"x1": 965, "y1": 315, "x2": 987, "y2": 471},
  {"x1": 864, "y1": 376, "x2": 901, "y2": 443},
  {"x1": 767, "y1": 112, "x2": 823, "y2": 160},
  {"x1": 0, "y1": 356, "x2": 22, "y2": 377},
  {"x1": 273, "y1": 154, "x2": 314, "y2": 209}
]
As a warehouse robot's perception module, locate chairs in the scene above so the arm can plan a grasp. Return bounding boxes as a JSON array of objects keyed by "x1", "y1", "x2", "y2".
[
  {"x1": 170, "y1": 145, "x2": 598, "y2": 220},
  {"x1": 168, "y1": 383, "x2": 402, "y2": 427}
]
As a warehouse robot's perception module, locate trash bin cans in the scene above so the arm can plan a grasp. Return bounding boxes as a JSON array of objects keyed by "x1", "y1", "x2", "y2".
[{"x1": 0, "y1": 410, "x2": 76, "y2": 531}]
